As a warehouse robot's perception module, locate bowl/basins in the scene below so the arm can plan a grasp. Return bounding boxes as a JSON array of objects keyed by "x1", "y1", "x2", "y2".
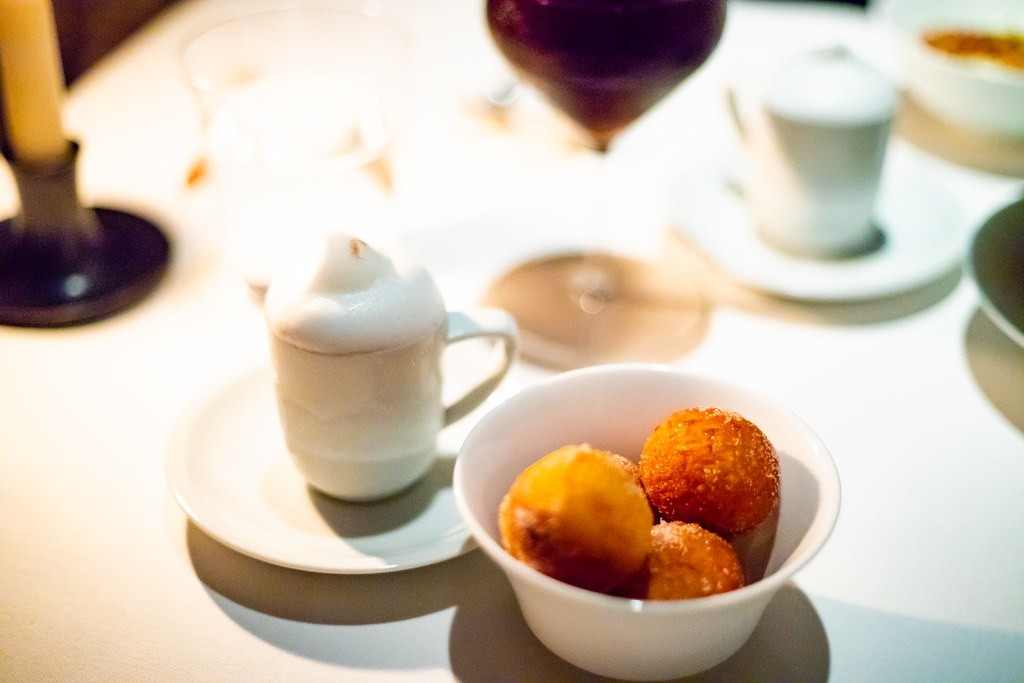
[
  {"x1": 452, "y1": 364, "x2": 841, "y2": 681},
  {"x1": 868, "y1": 0, "x2": 1024, "y2": 142},
  {"x1": 970, "y1": 192, "x2": 1024, "y2": 350}
]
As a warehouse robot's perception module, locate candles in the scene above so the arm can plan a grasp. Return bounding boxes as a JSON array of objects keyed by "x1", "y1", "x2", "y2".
[{"x1": 0, "y1": 0, "x2": 176, "y2": 331}]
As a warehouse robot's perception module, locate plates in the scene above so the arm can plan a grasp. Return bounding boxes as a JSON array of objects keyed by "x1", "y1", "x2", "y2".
[
  {"x1": 691, "y1": 156, "x2": 980, "y2": 300},
  {"x1": 166, "y1": 373, "x2": 518, "y2": 573}
]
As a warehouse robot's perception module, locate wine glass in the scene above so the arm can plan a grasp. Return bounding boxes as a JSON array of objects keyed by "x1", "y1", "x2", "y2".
[{"x1": 481, "y1": 0, "x2": 726, "y2": 370}]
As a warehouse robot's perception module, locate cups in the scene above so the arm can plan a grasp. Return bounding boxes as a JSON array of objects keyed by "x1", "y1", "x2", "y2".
[
  {"x1": 173, "y1": 11, "x2": 408, "y2": 304},
  {"x1": 728, "y1": 62, "x2": 894, "y2": 252},
  {"x1": 265, "y1": 237, "x2": 515, "y2": 505}
]
